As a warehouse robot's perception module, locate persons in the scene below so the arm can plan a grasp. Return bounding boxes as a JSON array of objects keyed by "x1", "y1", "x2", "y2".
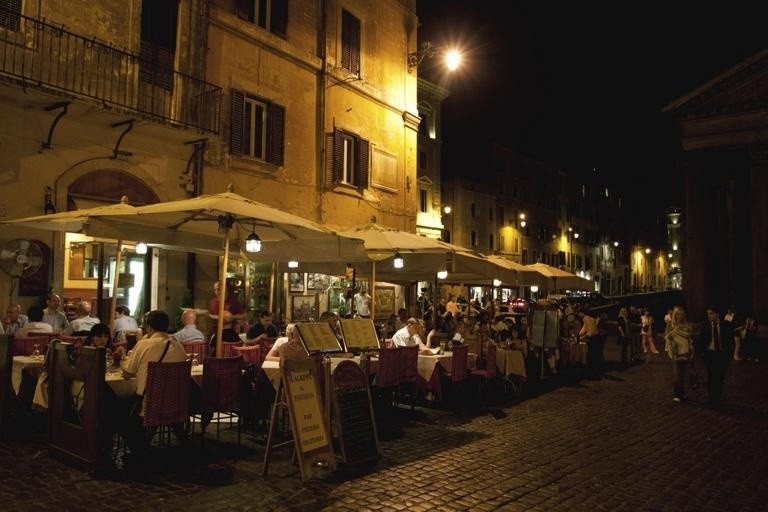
[
  {"x1": 617, "y1": 306, "x2": 660, "y2": 364},
  {"x1": 1, "y1": 280, "x2": 607, "y2": 444},
  {"x1": 695, "y1": 304, "x2": 759, "y2": 409},
  {"x1": 663, "y1": 304, "x2": 695, "y2": 403}
]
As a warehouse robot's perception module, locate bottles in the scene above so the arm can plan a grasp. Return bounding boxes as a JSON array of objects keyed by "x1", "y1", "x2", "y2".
[{"x1": 575, "y1": 332, "x2": 580, "y2": 345}]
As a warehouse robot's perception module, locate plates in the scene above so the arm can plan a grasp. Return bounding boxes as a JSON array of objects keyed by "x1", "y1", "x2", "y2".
[
  {"x1": 180, "y1": 307, "x2": 209, "y2": 317},
  {"x1": 206, "y1": 314, "x2": 237, "y2": 320}
]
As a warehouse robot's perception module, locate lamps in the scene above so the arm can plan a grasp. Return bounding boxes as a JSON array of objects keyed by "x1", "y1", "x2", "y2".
[
  {"x1": 393, "y1": 252, "x2": 404, "y2": 270},
  {"x1": 245, "y1": 222, "x2": 262, "y2": 254}
]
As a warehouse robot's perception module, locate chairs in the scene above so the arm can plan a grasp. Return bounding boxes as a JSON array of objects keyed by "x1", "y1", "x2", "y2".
[{"x1": 1, "y1": 315, "x2": 528, "y2": 474}]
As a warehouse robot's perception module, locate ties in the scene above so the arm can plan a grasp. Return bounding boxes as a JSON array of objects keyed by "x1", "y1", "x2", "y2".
[{"x1": 714, "y1": 322, "x2": 720, "y2": 353}]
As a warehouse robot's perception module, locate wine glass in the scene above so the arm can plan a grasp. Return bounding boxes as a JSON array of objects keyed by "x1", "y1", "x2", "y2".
[
  {"x1": 32, "y1": 344, "x2": 41, "y2": 357},
  {"x1": 192, "y1": 352, "x2": 199, "y2": 374},
  {"x1": 447, "y1": 341, "x2": 453, "y2": 355},
  {"x1": 185, "y1": 353, "x2": 192, "y2": 362}
]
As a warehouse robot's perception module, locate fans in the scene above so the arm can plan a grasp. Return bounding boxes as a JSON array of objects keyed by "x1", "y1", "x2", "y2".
[{"x1": 0, "y1": 239, "x2": 44, "y2": 296}]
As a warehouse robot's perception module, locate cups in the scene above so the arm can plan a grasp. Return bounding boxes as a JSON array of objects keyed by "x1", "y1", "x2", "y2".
[
  {"x1": 104, "y1": 353, "x2": 114, "y2": 373},
  {"x1": 440, "y1": 341, "x2": 445, "y2": 355}
]
